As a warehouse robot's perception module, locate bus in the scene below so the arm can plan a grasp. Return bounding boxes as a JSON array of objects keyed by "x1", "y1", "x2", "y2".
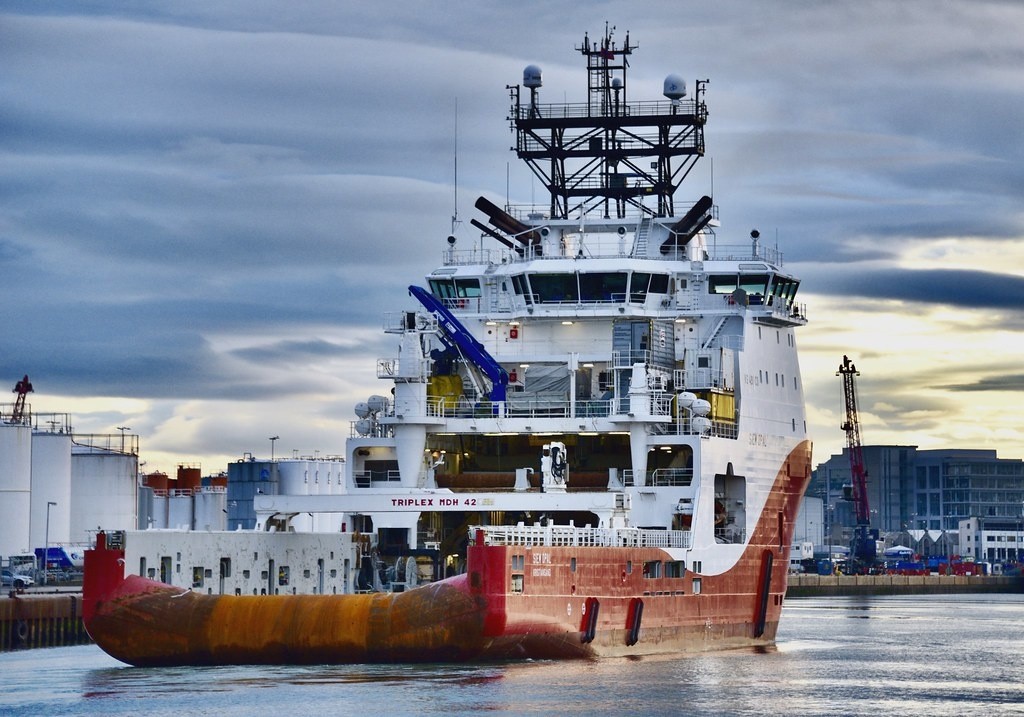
[{"x1": 0, "y1": 553, "x2": 38, "y2": 570}]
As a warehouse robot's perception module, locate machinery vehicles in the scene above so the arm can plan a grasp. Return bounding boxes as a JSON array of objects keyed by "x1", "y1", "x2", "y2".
[{"x1": 836, "y1": 354, "x2": 926, "y2": 574}]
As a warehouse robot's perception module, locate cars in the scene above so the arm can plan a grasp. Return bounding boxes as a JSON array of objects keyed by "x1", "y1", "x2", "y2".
[{"x1": 0, "y1": 569, "x2": 35, "y2": 589}]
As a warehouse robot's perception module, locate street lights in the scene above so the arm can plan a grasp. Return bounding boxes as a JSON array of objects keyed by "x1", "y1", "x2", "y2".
[{"x1": 43, "y1": 501, "x2": 58, "y2": 584}]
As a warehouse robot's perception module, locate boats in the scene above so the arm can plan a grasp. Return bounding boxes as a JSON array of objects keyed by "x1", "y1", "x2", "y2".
[{"x1": 80, "y1": 18, "x2": 815, "y2": 664}]
